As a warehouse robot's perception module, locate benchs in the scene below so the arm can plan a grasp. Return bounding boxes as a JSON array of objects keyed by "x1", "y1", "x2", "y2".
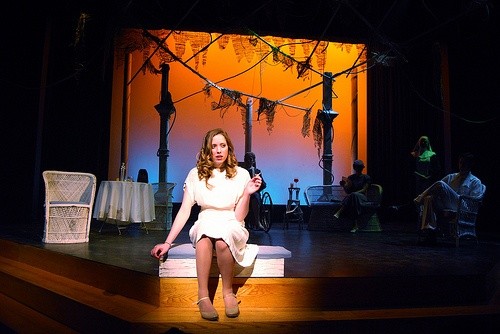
[{"x1": 302, "y1": 184, "x2": 382, "y2": 234}]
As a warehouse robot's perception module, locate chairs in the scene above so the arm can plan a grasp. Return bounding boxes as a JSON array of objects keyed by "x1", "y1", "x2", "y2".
[
  {"x1": 420, "y1": 181, "x2": 487, "y2": 250},
  {"x1": 143, "y1": 182, "x2": 177, "y2": 232},
  {"x1": 41, "y1": 170, "x2": 98, "y2": 244}
]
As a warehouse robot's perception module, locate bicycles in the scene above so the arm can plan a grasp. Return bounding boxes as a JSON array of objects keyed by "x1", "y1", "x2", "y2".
[{"x1": 247, "y1": 192, "x2": 273, "y2": 233}]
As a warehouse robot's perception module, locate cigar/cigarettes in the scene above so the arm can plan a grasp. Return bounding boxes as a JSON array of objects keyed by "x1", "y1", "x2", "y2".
[{"x1": 255, "y1": 171, "x2": 262, "y2": 178}]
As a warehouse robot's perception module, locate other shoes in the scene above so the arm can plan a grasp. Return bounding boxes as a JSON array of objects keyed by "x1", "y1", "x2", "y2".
[
  {"x1": 427, "y1": 224, "x2": 437, "y2": 230},
  {"x1": 350, "y1": 227, "x2": 359, "y2": 232},
  {"x1": 223, "y1": 294, "x2": 239, "y2": 317},
  {"x1": 334, "y1": 210, "x2": 341, "y2": 218},
  {"x1": 413, "y1": 195, "x2": 423, "y2": 204},
  {"x1": 197, "y1": 296, "x2": 218, "y2": 320}
]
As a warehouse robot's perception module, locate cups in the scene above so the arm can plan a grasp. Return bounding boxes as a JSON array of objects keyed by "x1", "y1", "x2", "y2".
[{"x1": 290, "y1": 183, "x2": 294, "y2": 187}]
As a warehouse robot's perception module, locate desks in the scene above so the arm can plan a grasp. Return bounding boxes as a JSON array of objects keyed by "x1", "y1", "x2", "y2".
[{"x1": 93, "y1": 180, "x2": 153, "y2": 238}]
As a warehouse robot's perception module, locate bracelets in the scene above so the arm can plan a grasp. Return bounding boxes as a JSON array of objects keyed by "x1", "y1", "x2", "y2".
[{"x1": 165, "y1": 241, "x2": 172, "y2": 248}]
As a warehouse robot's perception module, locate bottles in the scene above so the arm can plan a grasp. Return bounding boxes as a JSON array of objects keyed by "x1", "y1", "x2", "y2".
[{"x1": 119, "y1": 162, "x2": 126, "y2": 181}]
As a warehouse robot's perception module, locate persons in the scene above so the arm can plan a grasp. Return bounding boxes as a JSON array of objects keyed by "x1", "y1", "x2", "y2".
[
  {"x1": 151, "y1": 129, "x2": 262, "y2": 320},
  {"x1": 240, "y1": 152, "x2": 266, "y2": 230},
  {"x1": 334, "y1": 160, "x2": 370, "y2": 233},
  {"x1": 410, "y1": 136, "x2": 439, "y2": 171},
  {"x1": 414, "y1": 154, "x2": 483, "y2": 246}
]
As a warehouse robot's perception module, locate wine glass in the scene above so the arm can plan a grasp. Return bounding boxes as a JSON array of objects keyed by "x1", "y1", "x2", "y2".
[{"x1": 294, "y1": 177, "x2": 299, "y2": 188}]
